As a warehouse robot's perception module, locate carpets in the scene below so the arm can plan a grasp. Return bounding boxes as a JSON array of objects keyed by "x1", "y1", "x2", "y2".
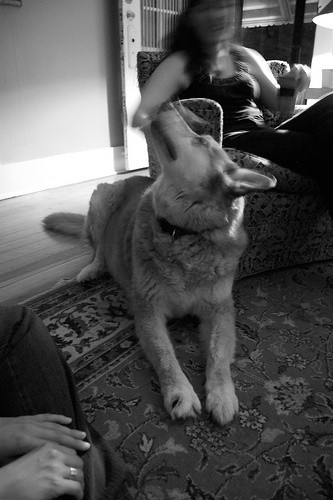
[{"x1": 12, "y1": 268, "x2": 333, "y2": 499}]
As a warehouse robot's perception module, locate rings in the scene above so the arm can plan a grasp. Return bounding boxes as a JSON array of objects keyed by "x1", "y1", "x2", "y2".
[{"x1": 69, "y1": 466, "x2": 76, "y2": 479}]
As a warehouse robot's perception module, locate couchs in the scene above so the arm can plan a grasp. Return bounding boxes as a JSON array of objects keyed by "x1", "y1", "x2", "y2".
[{"x1": 133, "y1": 52, "x2": 330, "y2": 284}]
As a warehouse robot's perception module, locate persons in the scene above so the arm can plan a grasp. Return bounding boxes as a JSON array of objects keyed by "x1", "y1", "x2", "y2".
[
  {"x1": 0, "y1": 412, "x2": 105, "y2": 500},
  {"x1": 133, "y1": 0, "x2": 332, "y2": 201}
]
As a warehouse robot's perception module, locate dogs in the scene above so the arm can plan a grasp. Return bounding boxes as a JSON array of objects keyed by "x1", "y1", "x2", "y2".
[{"x1": 42, "y1": 100, "x2": 277, "y2": 427}]
